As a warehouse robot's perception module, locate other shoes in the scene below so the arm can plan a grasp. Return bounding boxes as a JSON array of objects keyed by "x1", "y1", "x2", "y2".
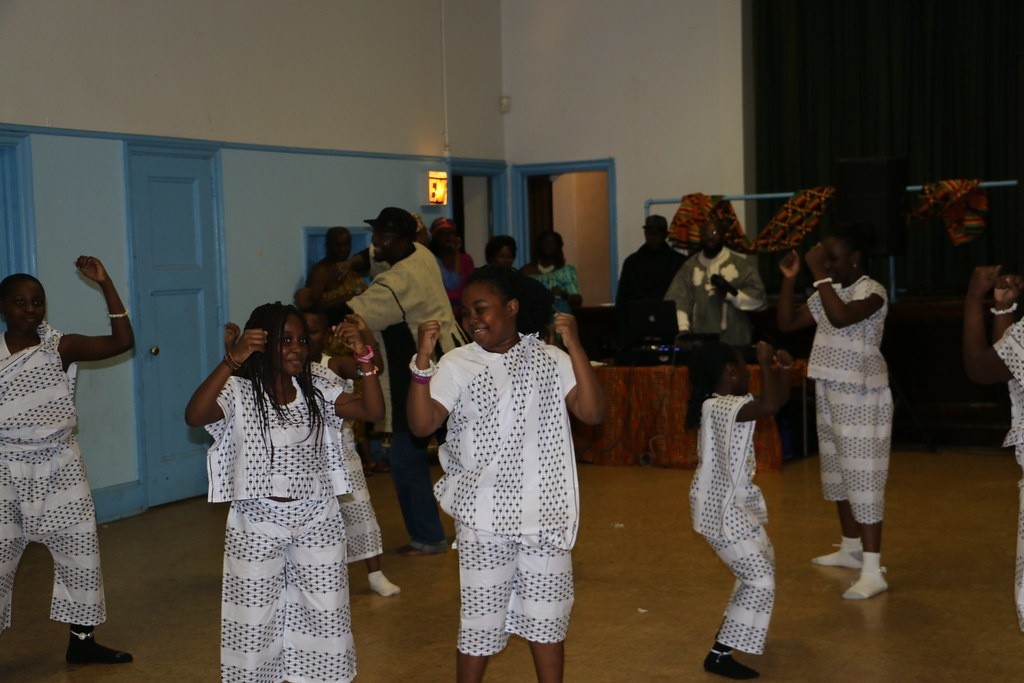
[
  {"x1": 398, "y1": 538, "x2": 449, "y2": 556},
  {"x1": 363, "y1": 465, "x2": 372, "y2": 478},
  {"x1": 368, "y1": 459, "x2": 389, "y2": 472}
]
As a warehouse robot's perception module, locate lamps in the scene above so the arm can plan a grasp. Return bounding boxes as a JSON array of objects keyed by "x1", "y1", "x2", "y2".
[{"x1": 419, "y1": 171, "x2": 449, "y2": 207}]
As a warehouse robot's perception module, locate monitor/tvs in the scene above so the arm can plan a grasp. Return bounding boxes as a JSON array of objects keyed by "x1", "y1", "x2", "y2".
[{"x1": 574, "y1": 300, "x2": 680, "y2": 362}]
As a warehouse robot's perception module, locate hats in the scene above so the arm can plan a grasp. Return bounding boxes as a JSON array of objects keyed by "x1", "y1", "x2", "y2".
[
  {"x1": 428, "y1": 218, "x2": 458, "y2": 236},
  {"x1": 363, "y1": 207, "x2": 416, "y2": 241},
  {"x1": 410, "y1": 212, "x2": 425, "y2": 233},
  {"x1": 642, "y1": 216, "x2": 667, "y2": 234}
]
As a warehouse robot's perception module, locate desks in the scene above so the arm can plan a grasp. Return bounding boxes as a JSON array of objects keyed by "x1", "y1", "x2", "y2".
[{"x1": 567, "y1": 358, "x2": 810, "y2": 469}]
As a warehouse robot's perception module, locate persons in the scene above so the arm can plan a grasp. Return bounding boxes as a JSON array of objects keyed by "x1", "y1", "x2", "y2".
[
  {"x1": 520, "y1": 231, "x2": 582, "y2": 349},
  {"x1": 335, "y1": 208, "x2": 468, "y2": 555},
  {"x1": 183, "y1": 302, "x2": 385, "y2": 682},
  {"x1": 224, "y1": 307, "x2": 401, "y2": 596},
  {"x1": 964, "y1": 266, "x2": 1024, "y2": 632},
  {"x1": 776, "y1": 221, "x2": 895, "y2": 601},
  {"x1": 431, "y1": 217, "x2": 475, "y2": 322},
  {"x1": 682, "y1": 342, "x2": 795, "y2": 678},
  {"x1": 405, "y1": 266, "x2": 605, "y2": 682},
  {"x1": 295, "y1": 227, "x2": 389, "y2": 476},
  {"x1": 486, "y1": 235, "x2": 516, "y2": 269},
  {"x1": 413, "y1": 212, "x2": 461, "y2": 288},
  {"x1": 605, "y1": 215, "x2": 767, "y2": 361},
  {"x1": 0, "y1": 256, "x2": 134, "y2": 665}
]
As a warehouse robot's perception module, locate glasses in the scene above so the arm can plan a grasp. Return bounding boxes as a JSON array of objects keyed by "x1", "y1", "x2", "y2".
[{"x1": 701, "y1": 230, "x2": 720, "y2": 236}]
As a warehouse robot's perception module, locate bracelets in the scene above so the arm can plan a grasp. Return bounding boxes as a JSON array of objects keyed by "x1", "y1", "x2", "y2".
[
  {"x1": 353, "y1": 345, "x2": 373, "y2": 363},
  {"x1": 228, "y1": 352, "x2": 242, "y2": 367},
  {"x1": 224, "y1": 355, "x2": 238, "y2": 370},
  {"x1": 108, "y1": 312, "x2": 127, "y2": 318},
  {"x1": 409, "y1": 353, "x2": 438, "y2": 383},
  {"x1": 990, "y1": 303, "x2": 1018, "y2": 316},
  {"x1": 356, "y1": 366, "x2": 379, "y2": 376},
  {"x1": 778, "y1": 362, "x2": 794, "y2": 369},
  {"x1": 813, "y1": 277, "x2": 832, "y2": 288}
]
